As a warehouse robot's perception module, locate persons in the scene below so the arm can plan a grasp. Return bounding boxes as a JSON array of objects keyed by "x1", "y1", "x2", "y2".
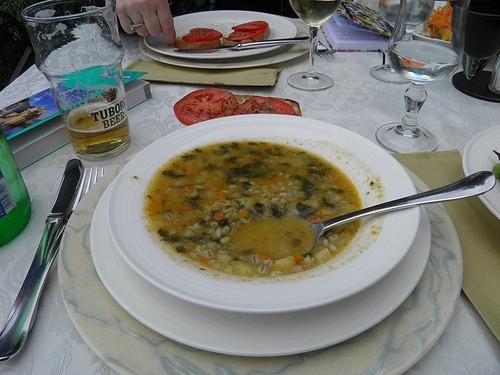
[{"x1": 115, "y1": 0, "x2": 176, "y2": 47}]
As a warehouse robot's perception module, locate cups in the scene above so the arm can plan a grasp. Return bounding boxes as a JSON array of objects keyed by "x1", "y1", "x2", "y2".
[
  {"x1": 449, "y1": 1, "x2": 500, "y2": 102},
  {"x1": 20, "y1": 0, "x2": 131, "y2": 161}
]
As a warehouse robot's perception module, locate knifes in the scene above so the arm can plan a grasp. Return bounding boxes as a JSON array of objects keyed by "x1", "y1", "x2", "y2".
[
  {"x1": 0, "y1": 158, "x2": 83, "y2": 361},
  {"x1": 173, "y1": 36, "x2": 312, "y2": 53}
]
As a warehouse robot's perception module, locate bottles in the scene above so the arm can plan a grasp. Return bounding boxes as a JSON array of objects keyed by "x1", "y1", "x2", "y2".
[{"x1": 0, "y1": 125, "x2": 33, "y2": 247}]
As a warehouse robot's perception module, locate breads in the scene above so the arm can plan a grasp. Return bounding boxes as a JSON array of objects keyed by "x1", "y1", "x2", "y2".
[
  {"x1": 223, "y1": 26, "x2": 270, "y2": 46},
  {"x1": 175, "y1": 35, "x2": 222, "y2": 49}
]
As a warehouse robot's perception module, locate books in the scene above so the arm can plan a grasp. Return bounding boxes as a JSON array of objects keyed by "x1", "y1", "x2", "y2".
[
  {"x1": 320, "y1": 0, "x2": 394, "y2": 52},
  {"x1": 0, "y1": 65, "x2": 153, "y2": 171}
]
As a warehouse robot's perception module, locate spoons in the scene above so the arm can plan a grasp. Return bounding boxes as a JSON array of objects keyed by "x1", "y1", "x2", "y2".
[{"x1": 281, "y1": 170, "x2": 496, "y2": 256}]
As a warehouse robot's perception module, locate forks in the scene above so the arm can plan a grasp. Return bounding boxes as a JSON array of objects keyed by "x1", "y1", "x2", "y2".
[{"x1": 71, "y1": 168, "x2": 104, "y2": 212}]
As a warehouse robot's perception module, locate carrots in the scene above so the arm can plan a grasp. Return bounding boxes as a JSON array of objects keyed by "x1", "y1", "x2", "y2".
[{"x1": 427, "y1": 3, "x2": 453, "y2": 40}]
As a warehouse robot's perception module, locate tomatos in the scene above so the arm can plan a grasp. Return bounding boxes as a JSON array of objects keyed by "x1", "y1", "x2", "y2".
[
  {"x1": 174, "y1": 89, "x2": 238, "y2": 125},
  {"x1": 232, "y1": 96, "x2": 298, "y2": 116},
  {"x1": 181, "y1": 21, "x2": 267, "y2": 41}
]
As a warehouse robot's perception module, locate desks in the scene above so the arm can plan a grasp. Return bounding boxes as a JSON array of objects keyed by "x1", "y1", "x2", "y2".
[{"x1": 0, "y1": 23, "x2": 500, "y2": 375}]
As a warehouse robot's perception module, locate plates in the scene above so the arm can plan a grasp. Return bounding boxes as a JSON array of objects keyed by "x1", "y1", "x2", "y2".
[
  {"x1": 462, "y1": 125, "x2": 500, "y2": 222},
  {"x1": 138, "y1": 18, "x2": 320, "y2": 69},
  {"x1": 108, "y1": 115, "x2": 421, "y2": 313},
  {"x1": 58, "y1": 161, "x2": 464, "y2": 375},
  {"x1": 89, "y1": 171, "x2": 431, "y2": 357},
  {"x1": 144, "y1": 11, "x2": 297, "y2": 59}
]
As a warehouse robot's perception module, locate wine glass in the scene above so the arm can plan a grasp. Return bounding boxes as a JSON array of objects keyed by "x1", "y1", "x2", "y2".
[
  {"x1": 370, "y1": 0, "x2": 411, "y2": 84},
  {"x1": 376, "y1": 0, "x2": 471, "y2": 152},
  {"x1": 287, "y1": 0, "x2": 341, "y2": 91}
]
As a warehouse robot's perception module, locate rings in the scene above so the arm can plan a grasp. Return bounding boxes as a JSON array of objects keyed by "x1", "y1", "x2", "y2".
[{"x1": 130, "y1": 23, "x2": 144, "y2": 30}]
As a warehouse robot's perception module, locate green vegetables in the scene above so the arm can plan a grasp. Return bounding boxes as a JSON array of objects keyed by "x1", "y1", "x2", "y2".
[{"x1": 146, "y1": 142, "x2": 344, "y2": 254}]
